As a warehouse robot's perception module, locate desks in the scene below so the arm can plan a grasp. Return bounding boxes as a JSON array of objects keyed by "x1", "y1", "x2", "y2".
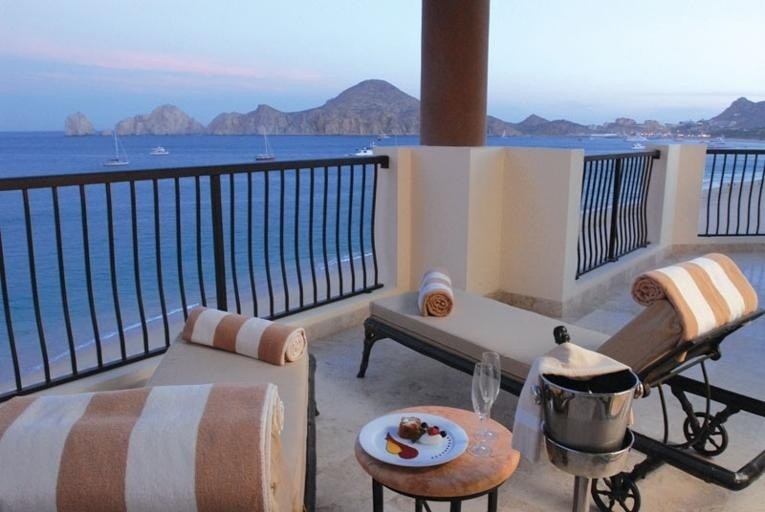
[{"x1": 354, "y1": 405, "x2": 520, "y2": 512}]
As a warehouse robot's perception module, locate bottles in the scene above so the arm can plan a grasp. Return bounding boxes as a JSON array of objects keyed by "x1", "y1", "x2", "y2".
[{"x1": 553, "y1": 326, "x2": 600, "y2": 393}]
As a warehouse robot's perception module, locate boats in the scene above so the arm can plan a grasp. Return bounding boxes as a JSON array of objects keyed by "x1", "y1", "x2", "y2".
[
  {"x1": 626, "y1": 134, "x2": 651, "y2": 149},
  {"x1": 674, "y1": 138, "x2": 684, "y2": 142},
  {"x1": 356, "y1": 138, "x2": 374, "y2": 156},
  {"x1": 699, "y1": 137, "x2": 725, "y2": 147},
  {"x1": 150, "y1": 147, "x2": 168, "y2": 155}
]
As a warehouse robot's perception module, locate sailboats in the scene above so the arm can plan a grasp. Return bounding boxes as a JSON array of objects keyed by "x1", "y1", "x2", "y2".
[
  {"x1": 104, "y1": 130, "x2": 130, "y2": 166},
  {"x1": 256, "y1": 130, "x2": 276, "y2": 160}
]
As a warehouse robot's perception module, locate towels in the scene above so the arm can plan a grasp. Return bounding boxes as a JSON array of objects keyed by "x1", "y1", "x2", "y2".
[
  {"x1": 181, "y1": 304, "x2": 308, "y2": 365},
  {"x1": 418, "y1": 265, "x2": 454, "y2": 317},
  {"x1": 510, "y1": 342, "x2": 636, "y2": 463}
]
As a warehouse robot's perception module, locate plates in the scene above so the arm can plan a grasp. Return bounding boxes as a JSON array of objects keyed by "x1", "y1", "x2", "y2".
[{"x1": 359, "y1": 413, "x2": 470, "y2": 467}]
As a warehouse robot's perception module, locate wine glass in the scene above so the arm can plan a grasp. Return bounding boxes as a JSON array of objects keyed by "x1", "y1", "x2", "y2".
[{"x1": 467, "y1": 352, "x2": 501, "y2": 457}]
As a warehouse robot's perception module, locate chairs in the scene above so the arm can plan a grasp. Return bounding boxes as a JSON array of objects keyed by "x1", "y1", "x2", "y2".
[
  {"x1": 1, "y1": 328, "x2": 317, "y2": 512},
  {"x1": 357, "y1": 284, "x2": 765, "y2": 512}
]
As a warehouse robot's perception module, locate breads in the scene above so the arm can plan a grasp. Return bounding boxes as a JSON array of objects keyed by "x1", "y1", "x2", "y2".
[{"x1": 399, "y1": 416, "x2": 421, "y2": 437}]
